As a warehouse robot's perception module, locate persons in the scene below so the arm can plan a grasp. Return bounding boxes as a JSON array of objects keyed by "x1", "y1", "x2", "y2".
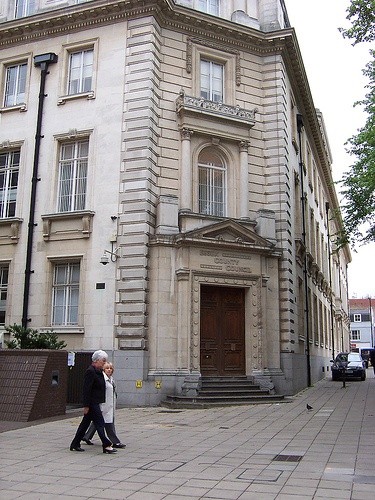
[
  {"x1": 70, "y1": 349, "x2": 118, "y2": 453},
  {"x1": 81, "y1": 361, "x2": 126, "y2": 448}
]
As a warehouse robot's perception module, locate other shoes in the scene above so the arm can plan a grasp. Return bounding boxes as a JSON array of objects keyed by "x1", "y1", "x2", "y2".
[
  {"x1": 113, "y1": 443, "x2": 126, "y2": 448},
  {"x1": 82, "y1": 437, "x2": 94, "y2": 445}
]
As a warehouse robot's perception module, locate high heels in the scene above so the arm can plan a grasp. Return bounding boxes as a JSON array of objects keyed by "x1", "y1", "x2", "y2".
[
  {"x1": 103, "y1": 448, "x2": 117, "y2": 453},
  {"x1": 70, "y1": 445, "x2": 85, "y2": 451}
]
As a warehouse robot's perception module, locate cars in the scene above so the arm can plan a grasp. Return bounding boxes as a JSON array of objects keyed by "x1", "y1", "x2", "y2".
[{"x1": 329, "y1": 351, "x2": 367, "y2": 381}]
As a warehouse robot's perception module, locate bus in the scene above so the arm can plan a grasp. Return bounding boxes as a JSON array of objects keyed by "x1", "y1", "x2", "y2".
[{"x1": 350, "y1": 347, "x2": 375, "y2": 368}]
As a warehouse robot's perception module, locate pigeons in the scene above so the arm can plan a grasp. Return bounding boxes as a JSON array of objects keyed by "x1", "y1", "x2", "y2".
[{"x1": 306, "y1": 403, "x2": 314, "y2": 411}]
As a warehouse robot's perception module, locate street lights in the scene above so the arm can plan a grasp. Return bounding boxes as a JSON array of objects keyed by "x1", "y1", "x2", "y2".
[{"x1": 367, "y1": 297, "x2": 373, "y2": 348}]
